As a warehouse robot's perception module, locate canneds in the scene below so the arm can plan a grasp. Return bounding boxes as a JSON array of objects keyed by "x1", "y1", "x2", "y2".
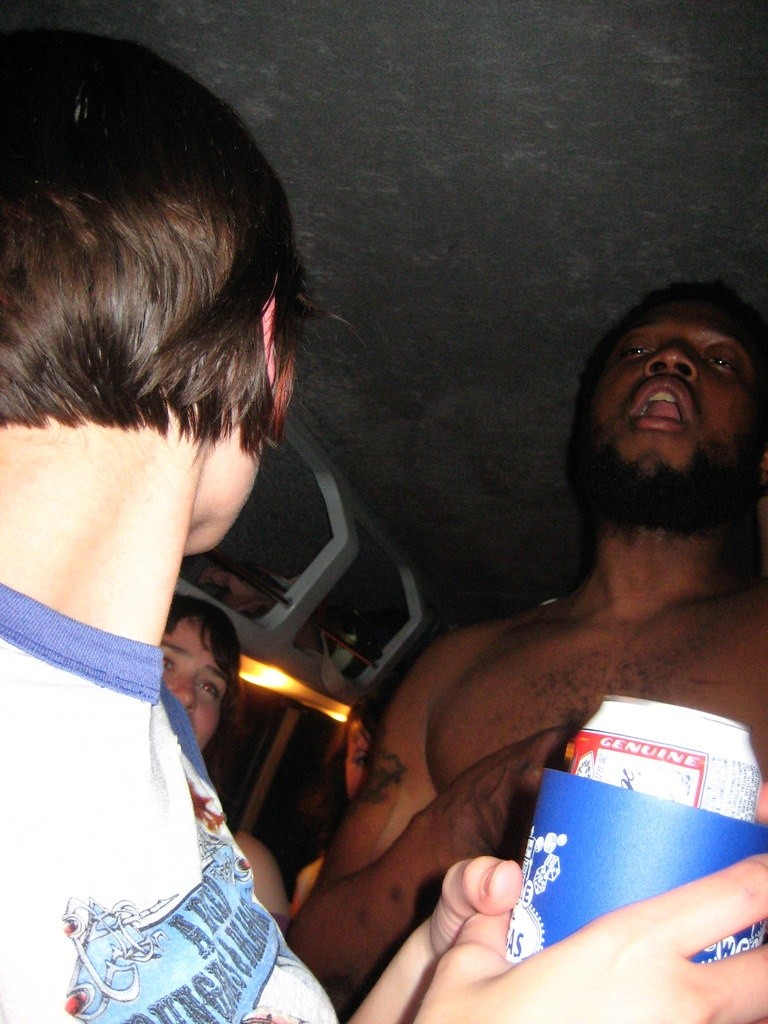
[{"x1": 564, "y1": 694, "x2": 762, "y2": 826}]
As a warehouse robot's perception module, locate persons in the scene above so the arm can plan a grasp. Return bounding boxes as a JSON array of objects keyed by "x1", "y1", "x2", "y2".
[
  {"x1": 288, "y1": 282, "x2": 768, "y2": 1022},
  {"x1": 289, "y1": 669, "x2": 406, "y2": 917},
  {"x1": 1, "y1": 25, "x2": 768, "y2": 1022},
  {"x1": 164, "y1": 595, "x2": 289, "y2": 936}
]
